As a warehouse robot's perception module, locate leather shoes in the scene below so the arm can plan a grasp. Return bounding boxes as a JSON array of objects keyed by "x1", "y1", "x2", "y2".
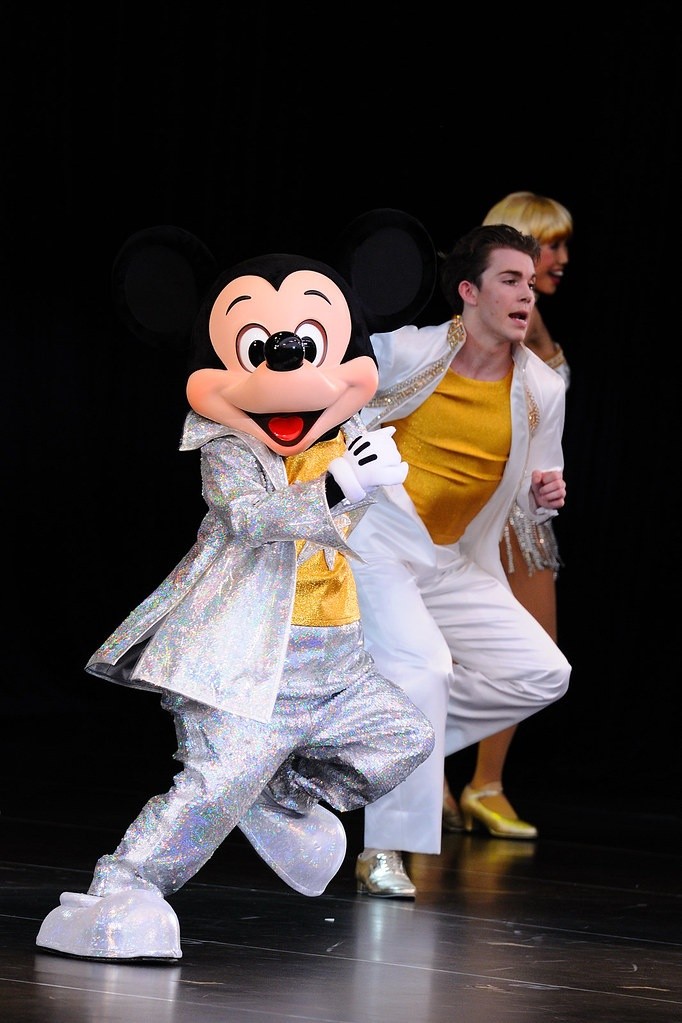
[{"x1": 355, "y1": 850, "x2": 417, "y2": 900}]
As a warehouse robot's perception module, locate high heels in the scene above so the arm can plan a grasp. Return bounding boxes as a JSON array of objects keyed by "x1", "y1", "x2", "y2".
[
  {"x1": 442, "y1": 787, "x2": 467, "y2": 833},
  {"x1": 461, "y1": 782, "x2": 537, "y2": 838}
]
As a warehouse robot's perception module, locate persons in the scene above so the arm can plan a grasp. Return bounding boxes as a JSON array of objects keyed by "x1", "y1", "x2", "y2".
[
  {"x1": 34, "y1": 208, "x2": 436, "y2": 961},
  {"x1": 349, "y1": 224, "x2": 570, "y2": 897},
  {"x1": 442, "y1": 193, "x2": 574, "y2": 836}
]
